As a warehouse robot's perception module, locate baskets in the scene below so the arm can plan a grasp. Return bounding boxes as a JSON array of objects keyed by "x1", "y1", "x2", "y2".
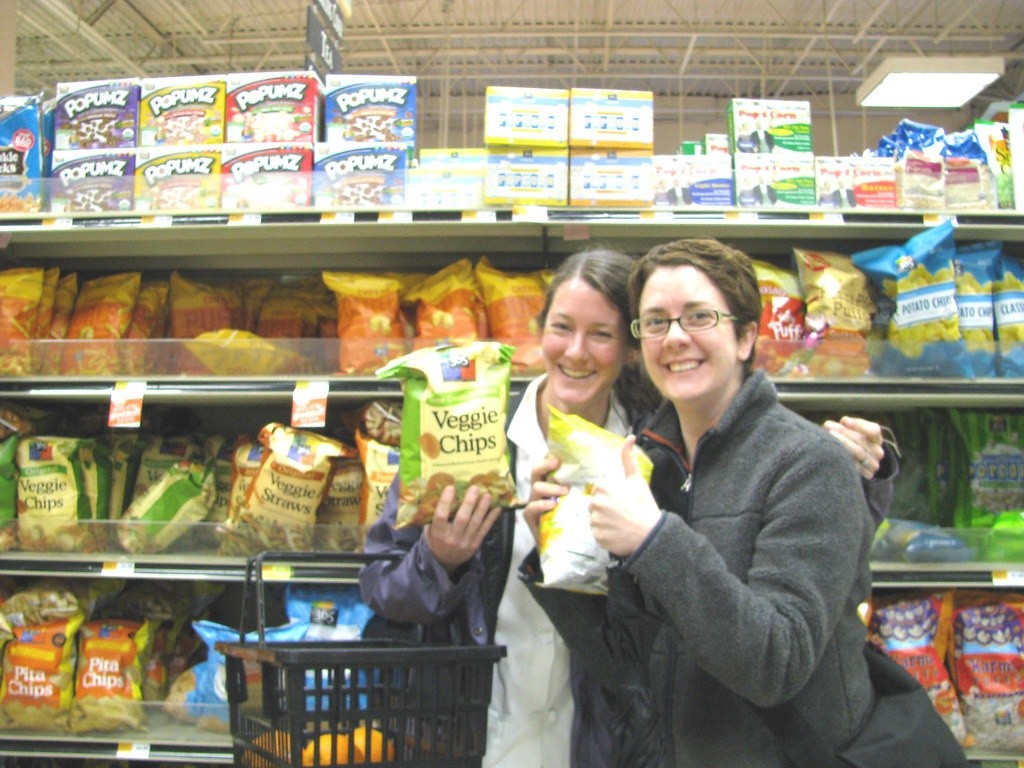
[{"x1": 213, "y1": 550, "x2": 507, "y2": 768}]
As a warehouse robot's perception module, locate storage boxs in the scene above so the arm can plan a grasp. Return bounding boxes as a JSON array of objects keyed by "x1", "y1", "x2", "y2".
[{"x1": 51, "y1": 71, "x2": 898, "y2": 210}]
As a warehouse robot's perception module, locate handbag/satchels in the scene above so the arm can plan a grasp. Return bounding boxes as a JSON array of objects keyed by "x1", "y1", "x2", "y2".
[{"x1": 833, "y1": 641, "x2": 970, "y2": 768}]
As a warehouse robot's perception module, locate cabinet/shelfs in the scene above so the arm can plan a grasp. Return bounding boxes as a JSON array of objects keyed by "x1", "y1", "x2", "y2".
[{"x1": 0, "y1": 206, "x2": 1024, "y2": 768}]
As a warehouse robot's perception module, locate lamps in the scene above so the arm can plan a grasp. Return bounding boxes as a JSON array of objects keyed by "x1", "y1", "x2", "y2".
[{"x1": 855, "y1": 53, "x2": 1006, "y2": 107}]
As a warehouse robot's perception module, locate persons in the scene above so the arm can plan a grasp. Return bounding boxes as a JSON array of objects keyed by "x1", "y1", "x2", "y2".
[
  {"x1": 655, "y1": 179, "x2": 693, "y2": 206},
  {"x1": 522, "y1": 237, "x2": 966, "y2": 768},
  {"x1": 356, "y1": 250, "x2": 901, "y2": 768},
  {"x1": 738, "y1": 119, "x2": 775, "y2": 154},
  {"x1": 817, "y1": 177, "x2": 858, "y2": 208},
  {"x1": 738, "y1": 174, "x2": 778, "y2": 207}
]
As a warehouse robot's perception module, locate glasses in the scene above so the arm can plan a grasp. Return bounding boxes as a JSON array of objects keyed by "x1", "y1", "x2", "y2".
[{"x1": 629, "y1": 308, "x2": 746, "y2": 339}]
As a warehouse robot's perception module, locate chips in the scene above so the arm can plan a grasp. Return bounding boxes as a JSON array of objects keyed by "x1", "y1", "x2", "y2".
[
  {"x1": 880, "y1": 263, "x2": 1024, "y2": 358},
  {"x1": 0, "y1": 693, "x2": 153, "y2": 736}
]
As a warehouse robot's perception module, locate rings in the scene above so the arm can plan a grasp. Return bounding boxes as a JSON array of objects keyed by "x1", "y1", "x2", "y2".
[{"x1": 861, "y1": 452, "x2": 871, "y2": 467}]
